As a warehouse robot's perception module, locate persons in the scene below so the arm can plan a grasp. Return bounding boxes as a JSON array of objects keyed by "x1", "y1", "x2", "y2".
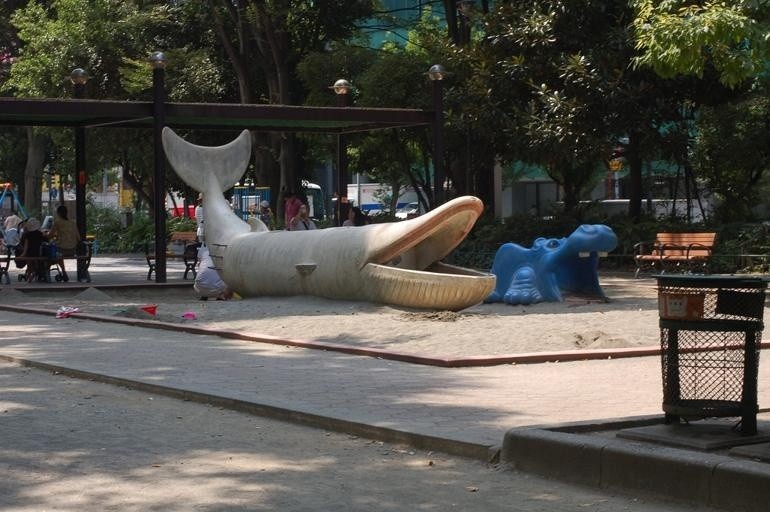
[
  {"x1": 188, "y1": 194, "x2": 319, "y2": 301},
  {"x1": 16, "y1": 217, "x2": 47, "y2": 274},
  {"x1": 2, "y1": 210, "x2": 24, "y2": 258},
  {"x1": 43, "y1": 206, "x2": 82, "y2": 280},
  {"x1": 342, "y1": 206, "x2": 368, "y2": 227}
]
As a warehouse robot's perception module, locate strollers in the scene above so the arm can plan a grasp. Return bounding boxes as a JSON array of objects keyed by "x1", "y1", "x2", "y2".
[{"x1": 13, "y1": 219, "x2": 63, "y2": 281}]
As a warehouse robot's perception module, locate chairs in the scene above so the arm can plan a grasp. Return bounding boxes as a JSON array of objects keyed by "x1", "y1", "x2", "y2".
[{"x1": 145, "y1": 239, "x2": 202, "y2": 280}]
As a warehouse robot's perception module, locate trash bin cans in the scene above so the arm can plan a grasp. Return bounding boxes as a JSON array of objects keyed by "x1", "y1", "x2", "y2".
[
  {"x1": 86, "y1": 236, "x2": 96, "y2": 257},
  {"x1": 657, "y1": 276, "x2": 766, "y2": 413}
]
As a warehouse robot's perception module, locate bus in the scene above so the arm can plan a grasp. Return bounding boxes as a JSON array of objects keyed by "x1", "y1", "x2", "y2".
[
  {"x1": 165, "y1": 180, "x2": 324, "y2": 225},
  {"x1": 42, "y1": 165, "x2": 150, "y2": 218}
]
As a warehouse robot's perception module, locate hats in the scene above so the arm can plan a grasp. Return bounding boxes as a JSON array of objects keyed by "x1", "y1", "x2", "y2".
[{"x1": 26, "y1": 217, "x2": 43, "y2": 232}]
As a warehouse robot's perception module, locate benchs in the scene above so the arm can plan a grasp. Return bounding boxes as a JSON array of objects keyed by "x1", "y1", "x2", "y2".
[
  {"x1": 632, "y1": 232, "x2": 718, "y2": 279},
  {"x1": 171, "y1": 231, "x2": 196, "y2": 243},
  {"x1": 0, "y1": 239, "x2": 93, "y2": 285}
]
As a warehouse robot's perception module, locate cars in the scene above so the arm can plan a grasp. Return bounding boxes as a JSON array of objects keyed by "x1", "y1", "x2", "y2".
[{"x1": 395, "y1": 201, "x2": 426, "y2": 220}]
